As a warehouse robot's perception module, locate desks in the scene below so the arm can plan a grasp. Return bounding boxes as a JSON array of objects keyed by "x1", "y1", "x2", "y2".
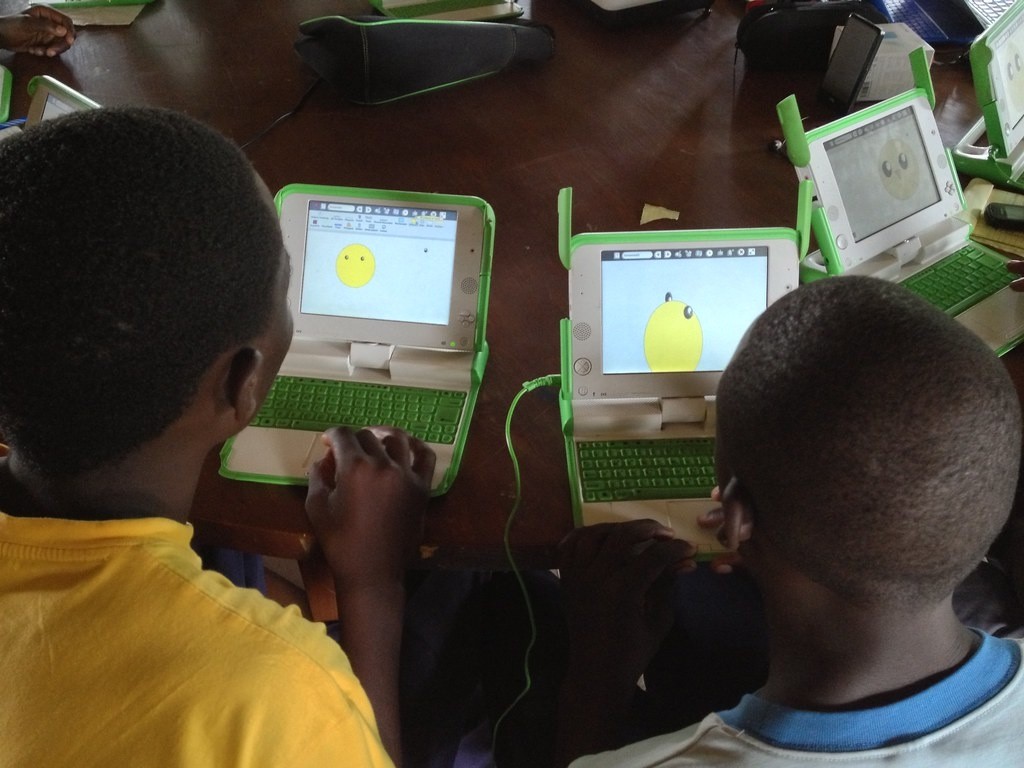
[{"x1": 9, "y1": 0, "x2": 978, "y2": 768}]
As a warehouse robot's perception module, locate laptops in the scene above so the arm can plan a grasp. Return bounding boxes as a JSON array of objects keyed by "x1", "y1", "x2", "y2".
[
  {"x1": 0, "y1": 0, "x2": 156, "y2": 132},
  {"x1": 214, "y1": 0, "x2": 1024, "y2": 558}
]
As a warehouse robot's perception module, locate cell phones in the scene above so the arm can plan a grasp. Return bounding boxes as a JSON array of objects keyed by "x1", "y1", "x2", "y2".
[
  {"x1": 984, "y1": 201, "x2": 1024, "y2": 232},
  {"x1": 812, "y1": 11, "x2": 883, "y2": 116}
]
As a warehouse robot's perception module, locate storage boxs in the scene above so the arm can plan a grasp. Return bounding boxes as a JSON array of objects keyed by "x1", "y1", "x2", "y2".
[{"x1": 829, "y1": 22, "x2": 935, "y2": 102}]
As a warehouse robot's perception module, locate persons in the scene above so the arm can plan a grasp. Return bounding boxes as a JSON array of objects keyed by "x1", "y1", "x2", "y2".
[
  {"x1": 559, "y1": 276, "x2": 1024, "y2": 768},
  {"x1": 0, "y1": 3, "x2": 77, "y2": 57},
  {"x1": 0, "y1": 105, "x2": 437, "y2": 768}
]
